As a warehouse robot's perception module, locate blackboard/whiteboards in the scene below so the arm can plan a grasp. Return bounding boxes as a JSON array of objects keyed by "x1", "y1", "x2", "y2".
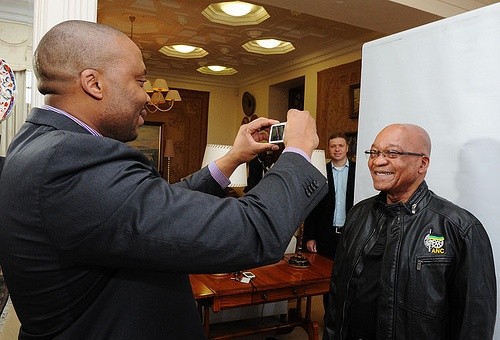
[{"x1": 349, "y1": 3, "x2": 499, "y2": 340}]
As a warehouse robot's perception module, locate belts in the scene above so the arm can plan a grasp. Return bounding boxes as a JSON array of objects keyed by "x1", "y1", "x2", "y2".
[{"x1": 333, "y1": 226, "x2": 343, "y2": 234}]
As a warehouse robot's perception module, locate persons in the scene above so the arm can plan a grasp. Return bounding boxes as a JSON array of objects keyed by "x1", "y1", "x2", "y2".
[
  {"x1": 0, "y1": 21, "x2": 330, "y2": 340},
  {"x1": 306, "y1": 132, "x2": 356, "y2": 324},
  {"x1": 242, "y1": 142, "x2": 285, "y2": 194},
  {"x1": 273, "y1": 128, "x2": 282, "y2": 141},
  {"x1": 323, "y1": 123, "x2": 497, "y2": 340}
]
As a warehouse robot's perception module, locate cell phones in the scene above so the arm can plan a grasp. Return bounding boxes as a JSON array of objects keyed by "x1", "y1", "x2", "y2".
[{"x1": 242, "y1": 272, "x2": 256, "y2": 278}]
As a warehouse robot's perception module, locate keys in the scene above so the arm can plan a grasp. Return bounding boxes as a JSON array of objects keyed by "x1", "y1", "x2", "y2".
[{"x1": 231, "y1": 276, "x2": 239, "y2": 281}]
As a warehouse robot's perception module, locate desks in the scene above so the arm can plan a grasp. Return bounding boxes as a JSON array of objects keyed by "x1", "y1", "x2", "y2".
[{"x1": 188, "y1": 250, "x2": 333, "y2": 340}]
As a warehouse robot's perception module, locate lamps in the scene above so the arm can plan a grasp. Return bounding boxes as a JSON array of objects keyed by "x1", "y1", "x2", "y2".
[
  {"x1": 128, "y1": 16, "x2": 182, "y2": 113},
  {"x1": 164, "y1": 139, "x2": 175, "y2": 183}
]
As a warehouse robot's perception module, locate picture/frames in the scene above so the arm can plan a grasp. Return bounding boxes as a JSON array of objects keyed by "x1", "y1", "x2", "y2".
[
  {"x1": 125, "y1": 121, "x2": 164, "y2": 177},
  {"x1": 347, "y1": 82, "x2": 360, "y2": 119}
]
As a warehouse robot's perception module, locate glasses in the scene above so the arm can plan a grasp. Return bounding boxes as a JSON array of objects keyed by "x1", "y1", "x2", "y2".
[{"x1": 364, "y1": 148, "x2": 424, "y2": 158}]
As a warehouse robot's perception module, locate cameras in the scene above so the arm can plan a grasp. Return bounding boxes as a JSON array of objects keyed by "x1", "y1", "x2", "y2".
[{"x1": 268, "y1": 122, "x2": 288, "y2": 144}]
